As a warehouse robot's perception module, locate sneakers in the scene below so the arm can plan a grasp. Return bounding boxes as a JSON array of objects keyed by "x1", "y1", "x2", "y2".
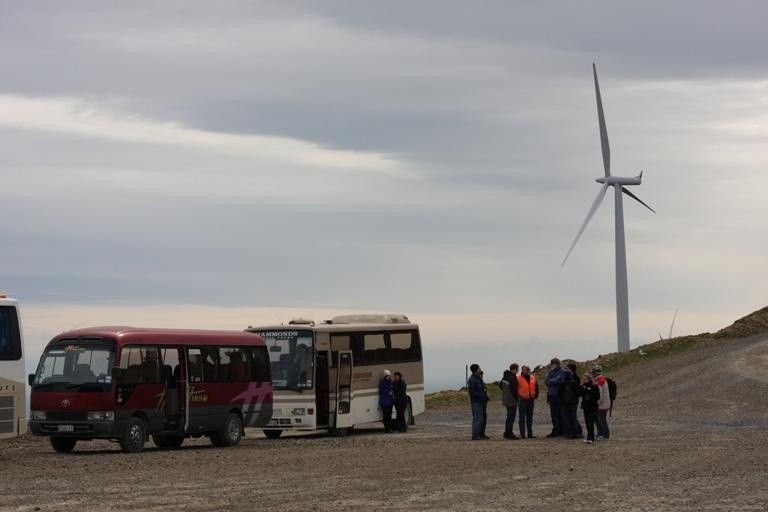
[
  {"x1": 595, "y1": 435, "x2": 609, "y2": 441},
  {"x1": 504, "y1": 432, "x2": 536, "y2": 440},
  {"x1": 473, "y1": 435, "x2": 490, "y2": 440},
  {"x1": 583, "y1": 440, "x2": 593, "y2": 444}
]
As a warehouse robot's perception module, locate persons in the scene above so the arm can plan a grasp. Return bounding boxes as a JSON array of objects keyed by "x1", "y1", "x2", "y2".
[
  {"x1": 468, "y1": 364, "x2": 486, "y2": 440},
  {"x1": 564, "y1": 363, "x2": 583, "y2": 438},
  {"x1": 545, "y1": 358, "x2": 566, "y2": 438},
  {"x1": 580, "y1": 374, "x2": 599, "y2": 444},
  {"x1": 227, "y1": 350, "x2": 247, "y2": 382},
  {"x1": 500, "y1": 363, "x2": 519, "y2": 440},
  {"x1": 592, "y1": 366, "x2": 611, "y2": 440},
  {"x1": 516, "y1": 366, "x2": 540, "y2": 438},
  {"x1": 478, "y1": 371, "x2": 490, "y2": 439},
  {"x1": 378, "y1": 369, "x2": 394, "y2": 433},
  {"x1": 393, "y1": 372, "x2": 407, "y2": 433},
  {"x1": 293, "y1": 342, "x2": 311, "y2": 385}
]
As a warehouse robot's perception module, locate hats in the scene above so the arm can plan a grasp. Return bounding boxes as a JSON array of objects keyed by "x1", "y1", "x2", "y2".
[
  {"x1": 592, "y1": 364, "x2": 602, "y2": 374},
  {"x1": 470, "y1": 364, "x2": 479, "y2": 372},
  {"x1": 383, "y1": 370, "x2": 391, "y2": 378}
]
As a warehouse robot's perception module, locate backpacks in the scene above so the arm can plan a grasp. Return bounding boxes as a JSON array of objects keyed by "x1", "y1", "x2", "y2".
[{"x1": 607, "y1": 378, "x2": 617, "y2": 400}]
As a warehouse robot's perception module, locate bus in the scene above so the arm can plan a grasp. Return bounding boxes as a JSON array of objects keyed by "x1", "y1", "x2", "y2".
[
  {"x1": 0, "y1": 292, "x2": 31, "y2": 440},
  {"x1": 241, "y1": 308, "x2": 427, "y2": 439},
  {"x1": 25, "y1": 325, "x2": 279, "y2": 456}
]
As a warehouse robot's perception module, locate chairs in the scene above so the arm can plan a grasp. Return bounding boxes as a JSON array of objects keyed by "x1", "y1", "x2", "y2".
[
  {"x1": 364, "y1": 347, "x2": 406, "y2": 360},
  {"x1": 123, "y1": 360, "x2": 222, "y2": 382}
]
